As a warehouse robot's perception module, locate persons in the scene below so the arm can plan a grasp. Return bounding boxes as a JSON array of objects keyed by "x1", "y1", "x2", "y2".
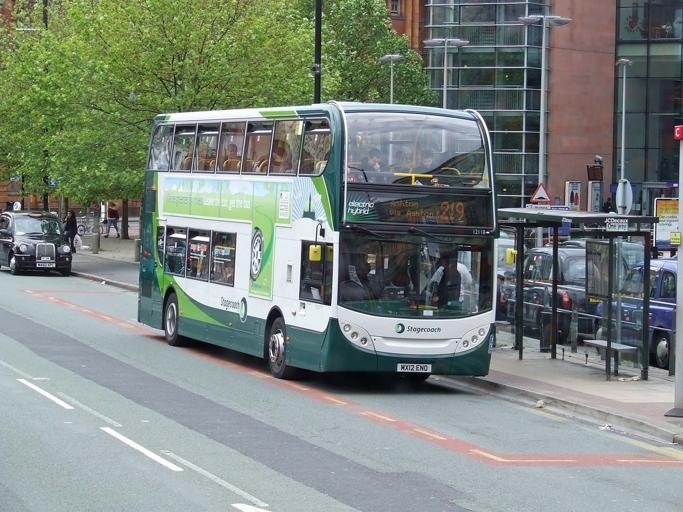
[
  {"x1": 218, "y1": 145, "x2": 240, "y2": 171},
  {"x1": 63, "y1": 209, "x2": 78, "y2": 254},
  {"x1": 158, "y1": 136, "x2": 185, "y2": 170},
  {"x1": 495, "y1": 274, "x2": 508, "y2": 321},
  {"x1": 603, "y1": 197, "x2": 614, "y2": 213},
  {"x1": 102, "y1": 201, "x2": 120, "y2": 239},
  {"x1": 337, "y1": 250, "x2": 479, "y2": 315},
  {"x1": 348, "y1": 131, "x2": 480, "y2": 192},
  {"x1": 524, "y1": 229, "x2": 534, "y2": 250}
]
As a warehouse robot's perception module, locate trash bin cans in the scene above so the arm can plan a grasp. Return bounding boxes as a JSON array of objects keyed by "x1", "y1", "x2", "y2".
[{"x1": 539, "y1": 310, "x2": 555, "y2": 353}]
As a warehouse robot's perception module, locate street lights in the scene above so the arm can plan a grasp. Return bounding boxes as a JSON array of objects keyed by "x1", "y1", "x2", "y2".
[
  {"x1": 376, "y1": 50, "x2": 406, "y2": 170},
  {"x1": 421, "y1": 36, "x2": 469, "y2": 160},
  {"x1": 612, "y1": 52, "x2": 635, "y2": 213},
  {"x1": 516, "y1": 14, "x2": 575, "y2": 204}
]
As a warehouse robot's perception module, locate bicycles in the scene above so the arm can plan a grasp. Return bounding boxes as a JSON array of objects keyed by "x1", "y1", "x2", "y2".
[{"x1": 76, "y1": 214, "x2": 105, "y2": 236}]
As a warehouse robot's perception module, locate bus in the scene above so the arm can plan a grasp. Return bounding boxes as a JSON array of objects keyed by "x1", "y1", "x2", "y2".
[{"x1": 132, "y1": 98, "x2": 501, "y2": 388}]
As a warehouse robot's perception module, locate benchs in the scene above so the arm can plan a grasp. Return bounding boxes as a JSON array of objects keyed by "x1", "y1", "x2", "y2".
[{"x1": 582, "y1": 340, "x2": 639, "y2": 378}]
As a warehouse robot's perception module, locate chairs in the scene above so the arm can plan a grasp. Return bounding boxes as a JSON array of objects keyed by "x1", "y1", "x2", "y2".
[{"x1": 180, "y1": 156, "x2": 279, "y2": 172}]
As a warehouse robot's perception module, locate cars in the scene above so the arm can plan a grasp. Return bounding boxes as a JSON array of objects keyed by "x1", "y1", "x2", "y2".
[
  {"x1": 498, "y1": 232, "x2": 678, "y2": 371},
  {"x1": 0, "y1": 210, "x2": 72, "y2": 276}
]
